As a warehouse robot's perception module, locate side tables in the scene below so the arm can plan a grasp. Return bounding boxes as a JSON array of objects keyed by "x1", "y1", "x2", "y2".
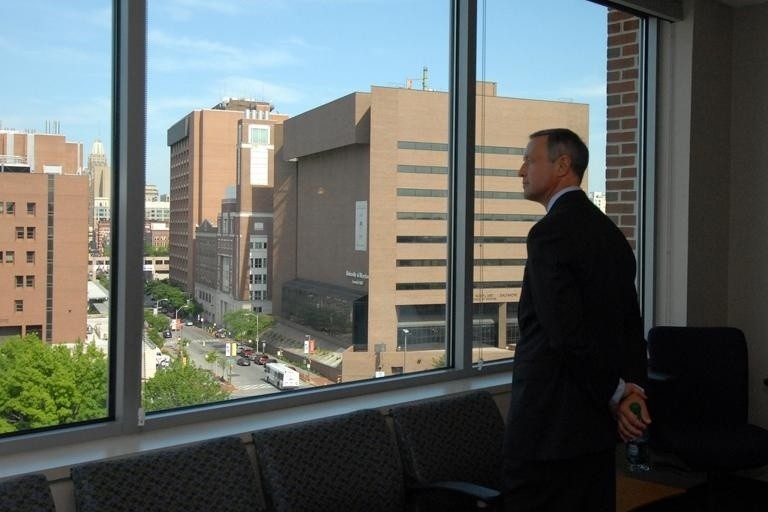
[{"x1": 616, "y1": 471, "x2": 685, "y2": 511}]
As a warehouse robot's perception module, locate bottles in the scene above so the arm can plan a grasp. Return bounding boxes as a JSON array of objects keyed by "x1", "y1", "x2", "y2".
[{"x1": 626, "y1": 402, "x2": 650, "y2": 475}]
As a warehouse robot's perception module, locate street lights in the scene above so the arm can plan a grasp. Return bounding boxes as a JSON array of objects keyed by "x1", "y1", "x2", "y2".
[
  {"x1": 245, "y1": 313, "x2": 259, "y2": 353},
  {"x1": 175, "y1": 305, "x2": 189, "y2": 337},
  {"x1": 402, "y1": 329, "x2": 409, "y2": 373},
  {"x1": 156, "y1": 298, "x2": 168, "y2": 317}
]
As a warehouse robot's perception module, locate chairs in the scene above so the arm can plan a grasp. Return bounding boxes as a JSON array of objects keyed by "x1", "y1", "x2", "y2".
[
  {"x1": 251, "y1": 406, "x2": 502, "y2": 512},
  {"x1": 1, "y1": 473, "x2": 56, "y2": 511},
  {"x1": 70, "y1": 436, "x2": 256, "y2": 512},
  {"x1": 639, "y1": 325, "x2": 766, "y2": 490},
  {"x1": 390, "y1": 390, "x2": 515, "y2": 502}
]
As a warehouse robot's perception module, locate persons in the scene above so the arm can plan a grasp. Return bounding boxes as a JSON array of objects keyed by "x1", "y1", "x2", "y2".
[{"x1": 494, "y1": 126, "x2": 653, "y2": 511}]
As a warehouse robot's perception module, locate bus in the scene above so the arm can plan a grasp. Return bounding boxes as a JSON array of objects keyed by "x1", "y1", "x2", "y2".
[{"x1": 265, "y1": 361, "x2": 299, "y2": 390}]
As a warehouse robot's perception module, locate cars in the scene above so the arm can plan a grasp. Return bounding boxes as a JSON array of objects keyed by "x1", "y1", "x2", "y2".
[
  {"x1": 187, "y1": 319, "x2": 193, "y2": 326},
  {"x1": 254, "y1": 355, "x2": 269, "y2": 365},
  {"x1": 215, "y1": 331, "x2": 226, "y2": 339},
  {"x1": 237, "y1": 357, "x2": 250, "y2": 366},
  {"x1": 163, "y1": 330, "x2": 172, "y2": 338},
  {"x1": 237, "y1": 343, "x2": 256, "y2": 360}
]
